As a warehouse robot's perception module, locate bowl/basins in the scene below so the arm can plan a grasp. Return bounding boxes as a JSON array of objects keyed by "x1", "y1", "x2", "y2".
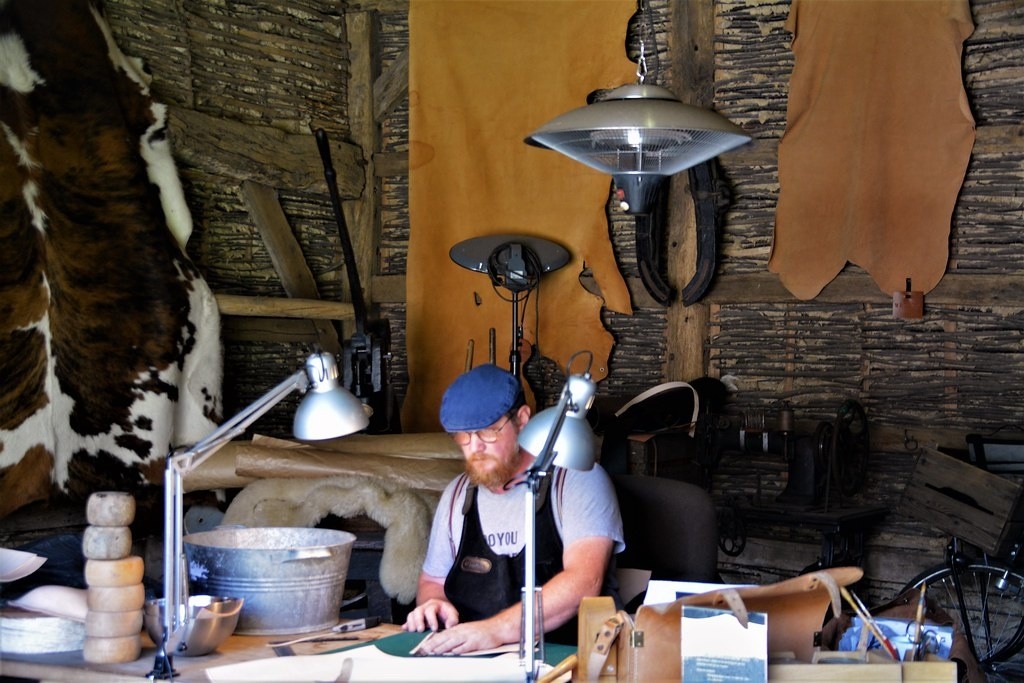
[{"x1": 143, "y1": 595, "x2": 245, "y2": 657}]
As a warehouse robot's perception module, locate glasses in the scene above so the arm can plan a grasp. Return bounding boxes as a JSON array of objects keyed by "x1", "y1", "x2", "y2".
[{"x1": 448, "y1": 416, "x2": 514, "y2": 445}]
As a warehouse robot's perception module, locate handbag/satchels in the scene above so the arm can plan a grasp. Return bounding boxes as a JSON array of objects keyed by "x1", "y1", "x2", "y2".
[{"x1": 601, "y1": 381, "x2": 712, "y2": 487}]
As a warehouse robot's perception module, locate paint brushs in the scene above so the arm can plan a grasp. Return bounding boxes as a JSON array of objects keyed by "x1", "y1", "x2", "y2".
[
  {"x1": 910, "y1": 580, "x2": 926, "y2": 660},
  {"x1": 851, "y1": 589, "x2": 896, "y2": 662},
  {"x1": 839, "y1": 586, "x2": 895, "y2": 660}
]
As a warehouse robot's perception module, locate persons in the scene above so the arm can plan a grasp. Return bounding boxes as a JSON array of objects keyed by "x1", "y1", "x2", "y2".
[{"x1": 401, "y1": 362, "x2": 625, "y2": 656}]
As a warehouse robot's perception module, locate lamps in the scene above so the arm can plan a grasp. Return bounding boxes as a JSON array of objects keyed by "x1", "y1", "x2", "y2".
[
  {"x1": 522, "y1": 0, "x2": 753, "y2": 219},
  {"x1": 144, "y1": 339, "x2": 371, "y2": 681},
  {"x1": 450, "y1": 233, "x2": 572, "y2": 376},
  {"x1": 502, "y1": 349, "x2": 598, "y2": 683}
]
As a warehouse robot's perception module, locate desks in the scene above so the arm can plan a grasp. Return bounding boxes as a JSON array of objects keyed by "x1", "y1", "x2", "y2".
[{"x1": 1, "y1": 608, "x2": 578, "y2": 683}]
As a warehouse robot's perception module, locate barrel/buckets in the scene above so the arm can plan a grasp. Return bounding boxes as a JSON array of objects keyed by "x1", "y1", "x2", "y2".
[{"x1": 181, "y1": 526, "x2": 357, "y2": 636}]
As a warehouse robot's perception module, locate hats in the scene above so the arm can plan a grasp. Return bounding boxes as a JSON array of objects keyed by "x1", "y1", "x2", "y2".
[{"x1": 439, "y1": 363, "x2": 519, "y2": 433}]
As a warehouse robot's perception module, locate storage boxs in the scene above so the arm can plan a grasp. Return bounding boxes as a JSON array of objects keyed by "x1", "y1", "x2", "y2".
[
  {"x1": 896, "y1": 443, "x2": 1024, "y2": 558},
  {"x1": 767, "y1": 647, "x2": 957, "y2": 683}
]
